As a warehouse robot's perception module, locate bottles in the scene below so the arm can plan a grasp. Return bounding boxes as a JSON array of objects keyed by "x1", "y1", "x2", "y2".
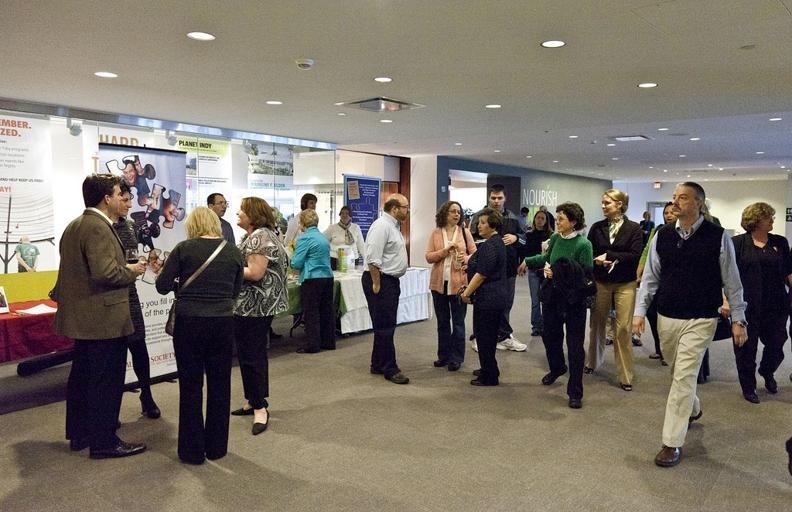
[{"x1": 337, "y1": 248, "x2": 356, "y2": 275}]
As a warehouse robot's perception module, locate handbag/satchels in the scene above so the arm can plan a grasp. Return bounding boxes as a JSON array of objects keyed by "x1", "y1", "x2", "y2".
[{"x1": 164, "y1": 299, "x2": 176, "y2": 336}]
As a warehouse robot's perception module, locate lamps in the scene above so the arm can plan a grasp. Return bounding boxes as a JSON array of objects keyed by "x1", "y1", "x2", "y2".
[
  {"x1": 164, "y1": 128, "x2": 178, "y2": 146},
  {"x1": 241, "y1": 138, "x2": 254, "y2": 155},
  {"x1": 64, "y1": 117, "x2": 82, "y2": 136}
]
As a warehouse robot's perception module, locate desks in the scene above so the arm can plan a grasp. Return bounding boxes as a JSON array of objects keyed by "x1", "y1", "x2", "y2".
[
  {"x1": 334, "y1": 266, "x2": 433, "y2": 336},
  {"x1": 0, "y1": 299, "x2": 77, "y2": 366},
  {"x1": 271, "y1": 278, "x2": 348, "y2": 354}
]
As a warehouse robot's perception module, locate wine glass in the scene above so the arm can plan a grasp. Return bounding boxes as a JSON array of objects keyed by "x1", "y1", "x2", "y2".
[{"x1": 125, "y1": 248, "x2": 140, "y2": 280}]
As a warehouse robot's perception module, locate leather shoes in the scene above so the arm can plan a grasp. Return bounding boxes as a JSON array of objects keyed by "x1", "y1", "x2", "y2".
[
  {"x1": 252, "y1": 410, "x2": 269, "y2": 435},
  {"x1": 70, "y1": 435, "x2": 91, "y2": 452},
  {"x1": 89, "y1": 435, "x2": 147, "y2": 459},
  {"x1": 231, "y1": 408, "x2": 254, "y2": 415},
  {"x1": 139, "y1": 393, "x2": 161, "y2": 419}
]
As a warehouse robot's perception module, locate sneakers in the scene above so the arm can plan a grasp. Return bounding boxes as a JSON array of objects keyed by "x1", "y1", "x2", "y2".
[
  {"x1": 653, "y1": 444, "x2": 683, "y2": 467},
  {"x1": 448, "y1": 360, "x2": 461, "y2": 371},
  {"x1": 740, "y1": 367, "x2": 778, "y2": 404},
  {"x1": 296, "y1": 342, "x2": 336, "y2": 355},
  {"x1": 542, "y1": 364, "x2": 568, "y2": 385},
  {"x1": 569, "y1": 397, "x2": 582, "y2": 409},
  {"x1": 433, "y1": 359, "x2": 448, "y2": 367},
  {"x1": 370, "y1": 365, "x2": 384, "y2": 374},
  {"x1": 583, "y1": 332, "x2": 669, "y2": 391},
  {"x1": 384, "y1": 369, "x2": 409, "y2": 384},
  {"x1": 472, "y1": 369, "x2": 501, "y2": 376},
  {"x1": 686, "y1": 409, "x2": 702, "y2": 430},
  {"x1": 471, "y1": 379, "x2": 499, "y2": 386},
  {"x1": 471, "y1": 329, "x2": 543, "y2": 352}
]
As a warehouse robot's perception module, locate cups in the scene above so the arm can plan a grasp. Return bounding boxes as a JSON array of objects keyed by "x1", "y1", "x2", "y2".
[{"x1": 450, "y1": 252, "x2": 463, "y2": 271}]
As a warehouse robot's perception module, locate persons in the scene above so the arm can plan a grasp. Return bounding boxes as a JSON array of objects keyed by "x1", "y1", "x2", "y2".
[
  {"x1": 322, "y1": 206, "x2": 365, "y2": 270},
  {"x1": 155, "y1": 206, "x2": 244, "y2": 465},
  {"x1": 291, "y1": 208, "x2": 336, "y2": 353},
  {"x1": 721, "y1": 203, "x2": 792, "y2": 403},
  {"x1": 283, "y1": 193, "x2": 317, "y2": 246},
  {"x1": 425, "y1": 200, "x2": 478, "y2": 372},
  {"x1": 361, "y1": 193, "x2": 410, "y2": 383},
  {"x1": 207, "y1": 193, "x2": 235, "y2": 244},
  {"x1": 15, "y1": 236, "x2": 39, "y2": 272},
  {"x1": 231, "y1": 196, "x2": 290, "y2": 435},
  {"x1": 110, "y1": 175, "x2": 160, "y2": 429},
  {"x1": 54, "y1": 173, "x2": 147, "y2": 460},
  {"x1": 122, "y1": 155, "x2": 179, "y2": 221},
  {"x1": 151, "y1": 256, "x2": 164, "y2": 273}
]
